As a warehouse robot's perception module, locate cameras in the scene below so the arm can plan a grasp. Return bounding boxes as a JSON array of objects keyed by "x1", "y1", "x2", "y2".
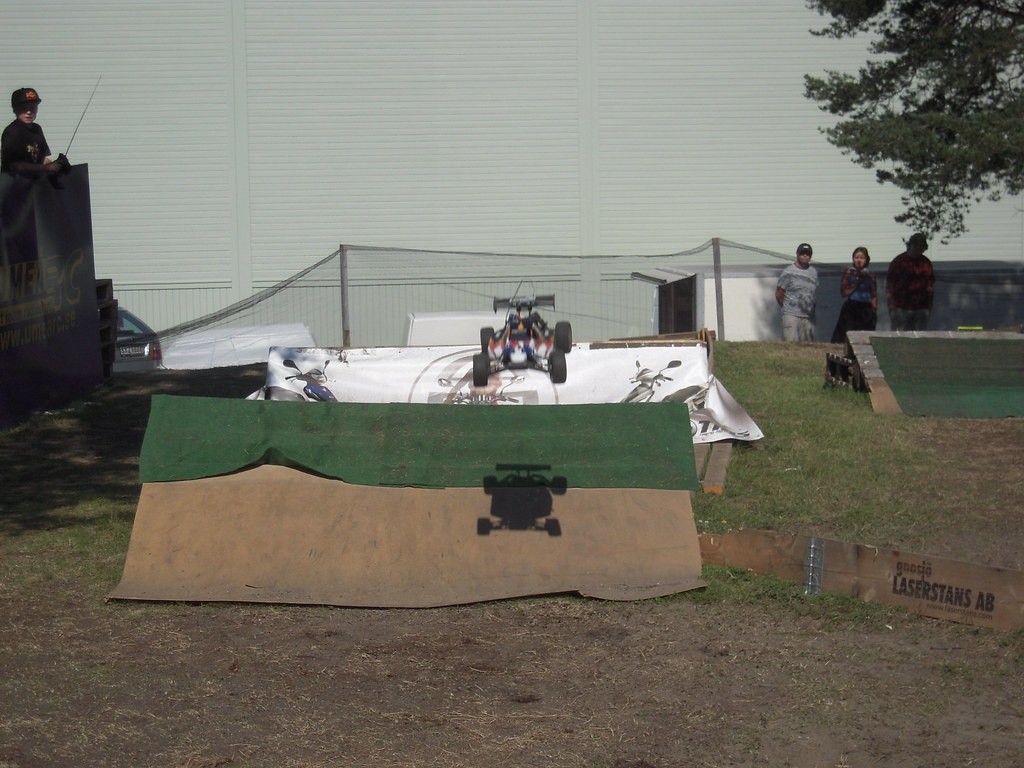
[{"x1": 849, "y1": 267, "x2": 857, "y2": 272}]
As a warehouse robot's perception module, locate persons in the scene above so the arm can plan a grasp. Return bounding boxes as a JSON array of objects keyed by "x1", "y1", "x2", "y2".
[
  {"x1": 831, "y1": 247, "x2": 878, "y2": 344},
  {"x1": 0, "y1": 88, "x2": 65, "y2": 174},
  {"x1": 885, "y1": 233, "x2": 935, "y2": 331},
  {"x1": 774, "y1": 243, "x2": 820, "y2": 344}
]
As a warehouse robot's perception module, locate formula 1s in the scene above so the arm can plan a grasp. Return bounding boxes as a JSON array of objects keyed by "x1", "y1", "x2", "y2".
[{"x1": 472, "y1": 293, "x2": 573, "y2": 388}]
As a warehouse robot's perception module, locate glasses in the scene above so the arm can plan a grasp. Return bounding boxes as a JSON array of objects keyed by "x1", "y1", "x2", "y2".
[{"x1": 799, "y1": 250, "x2": 811, "y2": 256}]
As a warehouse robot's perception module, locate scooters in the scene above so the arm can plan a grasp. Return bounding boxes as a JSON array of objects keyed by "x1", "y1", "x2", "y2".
[
  {"x1": 619, "y1": 359, "x2": 706, "y2": 436},
  {"x1": 264, "y1": 358, "x2": 339, "y2": 403},
  {"x1": 112, "y1": 306, "x2": 165, "y2": 370},
  {"x1": 438, "y1": 376, "x2": 525, "y2": 404}
]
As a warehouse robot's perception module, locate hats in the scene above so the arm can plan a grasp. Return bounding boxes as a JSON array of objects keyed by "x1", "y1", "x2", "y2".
[
  {"x1": 905, "y1": 233, "x2": 929, "y2": 251},
  {"x1": 797, "y1": 243, "x2": 812, "y2": 256}
]
